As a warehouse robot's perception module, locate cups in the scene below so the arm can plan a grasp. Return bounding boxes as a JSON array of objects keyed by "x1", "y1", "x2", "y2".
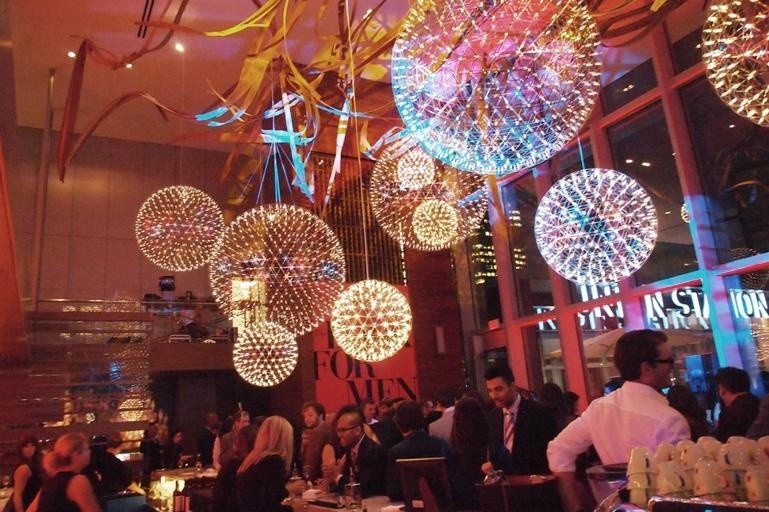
[{"x1": 625, "y1": 434, "x2": 768, "y2": 508}]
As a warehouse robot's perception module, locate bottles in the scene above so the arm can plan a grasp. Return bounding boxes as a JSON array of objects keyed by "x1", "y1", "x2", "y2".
[
  {"x1": 173, "y1": 480, "x2": 192, "y2": 512},
  {"x1": 345, "y1": 466, "x2": 363, "y2": 509},
  {"x1": 289, "y1": 463, "x2": 304, "y2": 502}
]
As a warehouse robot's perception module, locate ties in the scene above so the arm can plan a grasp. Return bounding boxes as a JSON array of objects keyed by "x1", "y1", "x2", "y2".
[
  {"x1": 504, "y1": 409, "x2": 516, "y2": 455},
  {"x1": 349, "y1": 448, "x2": 357, "y2": 484}
]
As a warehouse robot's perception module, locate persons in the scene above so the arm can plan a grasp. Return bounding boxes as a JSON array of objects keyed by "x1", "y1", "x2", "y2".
[
  {"x1": 299, "y1": 363, "x2": 622, "y2": 510},
  {"x1": 193, "y1": 411, "x2": 306, "y2": 510},
  {"x1": 13, "y1": 431, "x2": 133, "y2": 510},
  {"x1": 544, "y1": 329, "x2": 693, "y2": 511},
  {"x1": 138, "y1": 426, "x2": 183, "y2": 475},
  {"x1": 666, "y1": 366, "x2": 767, "y2": 443}
]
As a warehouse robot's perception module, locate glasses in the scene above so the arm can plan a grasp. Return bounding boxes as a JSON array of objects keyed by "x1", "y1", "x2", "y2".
[
  {"x1": 639, "y1": 358, "x2": 674, "y2": 365},
  {"x1": 336, "y1": 425, "x2": 360, "y2": 432}
]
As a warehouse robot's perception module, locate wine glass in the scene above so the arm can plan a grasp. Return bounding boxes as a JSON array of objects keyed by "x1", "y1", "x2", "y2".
[{"x1": 2, "y1": 476, "x2": 10, "y2": 492}]
